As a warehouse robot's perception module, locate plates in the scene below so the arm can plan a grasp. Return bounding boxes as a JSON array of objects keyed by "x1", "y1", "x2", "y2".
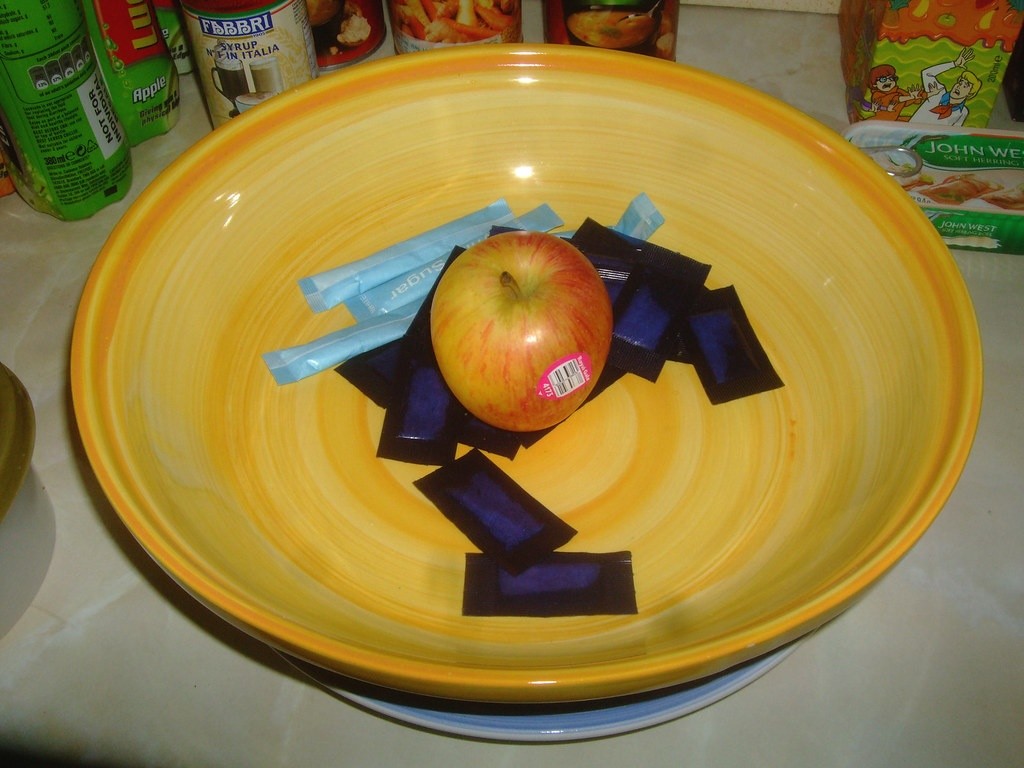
[{"x1": 275, "y1": 632, "x2": 810, "y2": 741}]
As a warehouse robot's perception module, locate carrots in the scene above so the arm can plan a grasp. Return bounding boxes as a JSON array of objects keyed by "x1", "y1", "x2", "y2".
[{"x1": 401, "y1": 0, "x2": 518, "y2": 43}]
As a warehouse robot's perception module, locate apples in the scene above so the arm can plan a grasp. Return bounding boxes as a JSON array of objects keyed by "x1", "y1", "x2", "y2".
[{"x1": 430, "y1": 230, "x2": 614, "y2": 432}]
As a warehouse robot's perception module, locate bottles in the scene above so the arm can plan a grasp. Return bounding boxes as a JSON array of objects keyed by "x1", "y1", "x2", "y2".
[{"x1": 0, "y1": 0, "x2": 682, "y2": 222}]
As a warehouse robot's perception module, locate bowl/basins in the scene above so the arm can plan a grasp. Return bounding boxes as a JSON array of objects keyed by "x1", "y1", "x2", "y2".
[{"x1": 69, "y1": 42, "x2": 983, "y2": 704}]
